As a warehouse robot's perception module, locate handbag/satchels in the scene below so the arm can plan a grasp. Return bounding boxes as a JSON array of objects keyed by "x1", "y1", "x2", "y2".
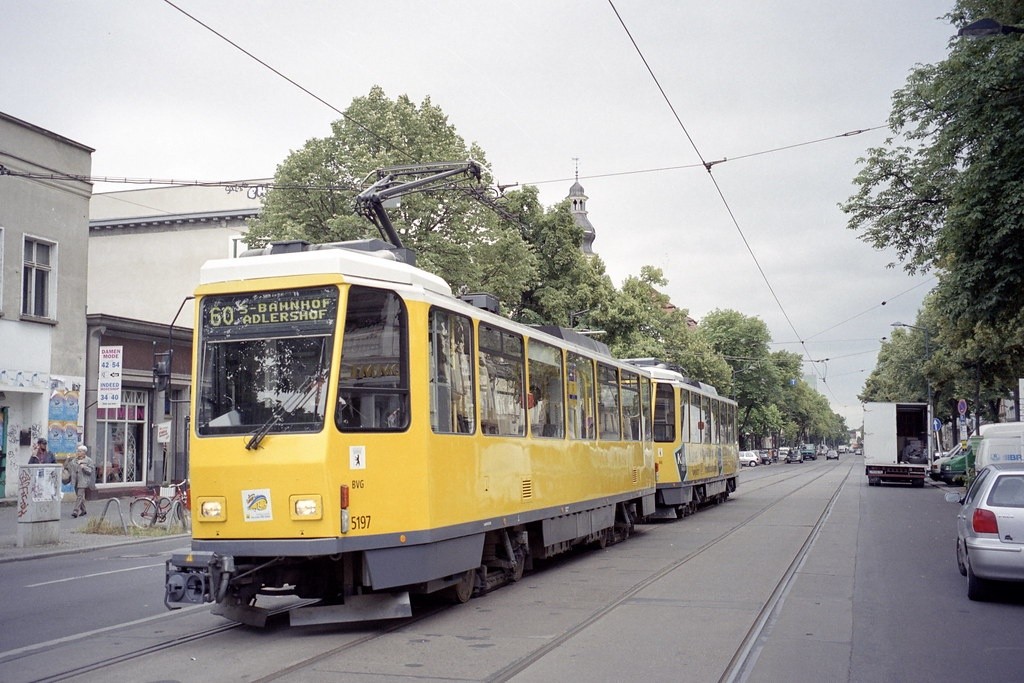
[{"x1": 62, "y1": 469, "x2": 71, "y2": 484}]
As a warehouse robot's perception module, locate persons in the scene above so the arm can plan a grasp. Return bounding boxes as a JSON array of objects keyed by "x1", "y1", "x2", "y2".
[
  {"x1": 28, "y1": 439, "x2": 56, "y2": 463},
  {"x1": 64, "y1": 446, "x2": 94, "y2": 518},
  {"x1": 112, "y1": 445, "x2": 123, "y2": 482}
]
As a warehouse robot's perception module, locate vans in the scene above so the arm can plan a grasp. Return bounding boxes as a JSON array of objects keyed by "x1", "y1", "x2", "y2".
[{"x1": 931, "y1": 422, "x2": 1024, "y2": 489}]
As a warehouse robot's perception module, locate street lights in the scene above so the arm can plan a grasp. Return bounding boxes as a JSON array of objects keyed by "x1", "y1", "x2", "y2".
[{"x1": 889, "y1": 321, "x2": 932, "y2": 404}]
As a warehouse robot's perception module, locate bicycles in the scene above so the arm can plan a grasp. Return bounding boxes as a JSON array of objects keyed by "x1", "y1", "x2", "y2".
[{"x1": 128, "y1": 478, "x2": 188, "y2": 531}]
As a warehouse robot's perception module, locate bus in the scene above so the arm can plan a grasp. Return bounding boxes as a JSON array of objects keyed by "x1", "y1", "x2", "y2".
[{"x1": 152, "y1": 153, "x2": 740, "y2": 627}]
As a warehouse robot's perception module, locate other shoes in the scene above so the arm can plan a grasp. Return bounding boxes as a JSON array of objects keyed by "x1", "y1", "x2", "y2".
[
  {"x1": 79, "y1": 511, "x2": 87, "y2": 516},
  {"x1": 71, "y1": 513, "x2": 78, "y2": 518}
]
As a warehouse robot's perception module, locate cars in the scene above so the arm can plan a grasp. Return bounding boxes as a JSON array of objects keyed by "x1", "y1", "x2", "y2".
[
  {"x1": 739, "y1": 444, "x2": 862, "y2": 467},
  {"x1": 945, "y1": 461, "x2": 1024, "y2": 602}
]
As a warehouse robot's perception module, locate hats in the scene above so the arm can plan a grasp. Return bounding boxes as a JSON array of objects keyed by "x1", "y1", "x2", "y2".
[{"x1": 37, "y1": 438, "x2": 47, "y2": 444}]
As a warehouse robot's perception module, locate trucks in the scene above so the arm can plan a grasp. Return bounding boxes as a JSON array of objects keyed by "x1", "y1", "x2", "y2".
[{"x1": 863, "y1": 402, "x2": 931, "y2": 489}]
